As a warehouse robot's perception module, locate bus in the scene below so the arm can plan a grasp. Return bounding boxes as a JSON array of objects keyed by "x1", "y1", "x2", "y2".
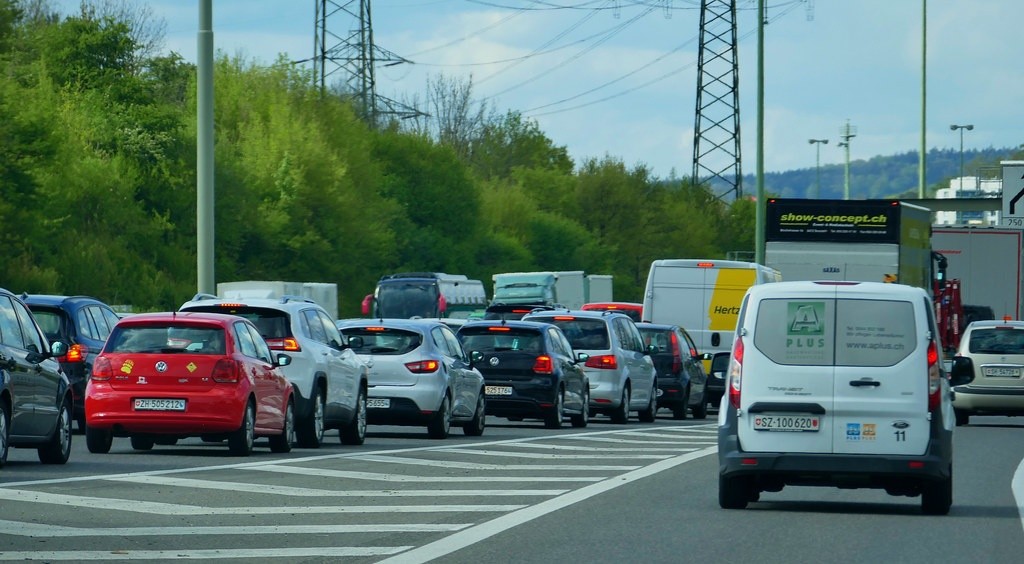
[{"x1": 357, "y1": 272, "x2": 488, "y2": 322}]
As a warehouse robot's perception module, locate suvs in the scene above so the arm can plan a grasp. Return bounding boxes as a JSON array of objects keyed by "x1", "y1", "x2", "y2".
[
  {"x1": 630, "y1": 323, "x2": 713, "y2": 420},
  {"x1": 12, "y1": 294, "x2": 119, "y2": 432},
  {"x1": 177, "y1": 291, "x2": 369, "y2": 445},
  {"x1": 520, "y1": 306, "x2": 659, "y2": 424}
]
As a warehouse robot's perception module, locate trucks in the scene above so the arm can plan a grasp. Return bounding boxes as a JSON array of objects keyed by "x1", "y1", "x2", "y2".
[
  {"x1": 762, "y1": 194, "x2": 948, "y2": 292},
  {"x1": 486, "y1": 271, "x2": 589, "y2": 317}
]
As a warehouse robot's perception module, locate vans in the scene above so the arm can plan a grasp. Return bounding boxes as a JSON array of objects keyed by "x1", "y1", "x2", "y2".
[
  {"x1": 580, "y1": 302, "x2": 644, "y2": 322},
  {"x1": 640, "y1": 258, "x2": 783, "y2": 378},
  {"x1": 713, "y1": 280, "x2": 975, "y2": 519}
]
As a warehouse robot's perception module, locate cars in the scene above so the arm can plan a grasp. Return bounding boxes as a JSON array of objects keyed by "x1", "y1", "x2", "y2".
[
  {"x1": 948, "y1": 314, "x2": 1024, "y2": 426},
  {"x1": 448, "y1": 320, "x2": 593, "y2": 428},
  {"x1": 0, "y1": 288, "x2": 76, "y2": 464},
  {"x1": 709, "y1": 350, "x2": 734, "y2": 410},
  {"x1": 333, "y1": 318, "x2": 489, "y2": 439},
  {"x1": 79, "y1": 312, "x2": 297, "y2": 456}
]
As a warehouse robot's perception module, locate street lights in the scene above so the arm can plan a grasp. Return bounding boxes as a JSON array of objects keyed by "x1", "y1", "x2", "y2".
[
  {"x1": 806, "y1": 139, "x2": 828, "y2": 198},
  {"x1": 949, "y1": 124, "x2": 973, "y2": 225}
]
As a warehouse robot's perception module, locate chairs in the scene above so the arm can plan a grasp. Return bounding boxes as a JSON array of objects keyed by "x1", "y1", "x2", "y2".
[{"x1": 114, "y1": 336, "x2": 668, "y2": 356}]
